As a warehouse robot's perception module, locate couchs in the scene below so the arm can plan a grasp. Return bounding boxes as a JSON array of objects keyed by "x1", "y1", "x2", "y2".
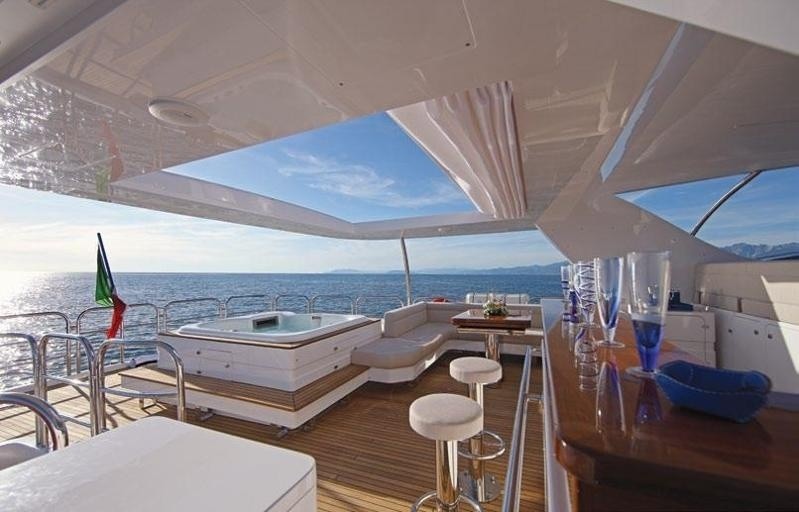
[{"x1": 350, "y1": 301, "x2": 546, "y2": 390}]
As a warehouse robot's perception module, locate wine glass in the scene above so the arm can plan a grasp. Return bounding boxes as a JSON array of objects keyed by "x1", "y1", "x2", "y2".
[{"x1": 560, "y1": 250, "x2": 672, "y2": 385}]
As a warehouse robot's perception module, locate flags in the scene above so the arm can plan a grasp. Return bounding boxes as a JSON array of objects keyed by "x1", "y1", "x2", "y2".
[{"x1": 94, "y1": 238, "x2": 126, "y2": 339}]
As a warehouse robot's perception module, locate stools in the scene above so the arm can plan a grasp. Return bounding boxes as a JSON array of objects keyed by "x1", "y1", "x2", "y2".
[{"x1": 408, "y1": 357, "x2": 506, "y2": 512}]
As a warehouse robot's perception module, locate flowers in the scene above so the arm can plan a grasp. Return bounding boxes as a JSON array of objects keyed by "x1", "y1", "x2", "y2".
[{"x1": 482, "y1": 297, "x2": 509, "y2": 316}]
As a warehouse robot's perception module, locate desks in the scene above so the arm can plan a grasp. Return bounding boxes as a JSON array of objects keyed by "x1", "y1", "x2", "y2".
[
  {"x1": 451, "y1": 308, "x2": 533, "y2": 385},
  {"x1": 0, "y1": 415, "x2": 317, "y2": 512}
]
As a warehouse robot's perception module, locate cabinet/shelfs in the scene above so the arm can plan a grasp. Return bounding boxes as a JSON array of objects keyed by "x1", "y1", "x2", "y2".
[{"x1": 652, "y1": 300, "x2": 799, "y2": 396}]
